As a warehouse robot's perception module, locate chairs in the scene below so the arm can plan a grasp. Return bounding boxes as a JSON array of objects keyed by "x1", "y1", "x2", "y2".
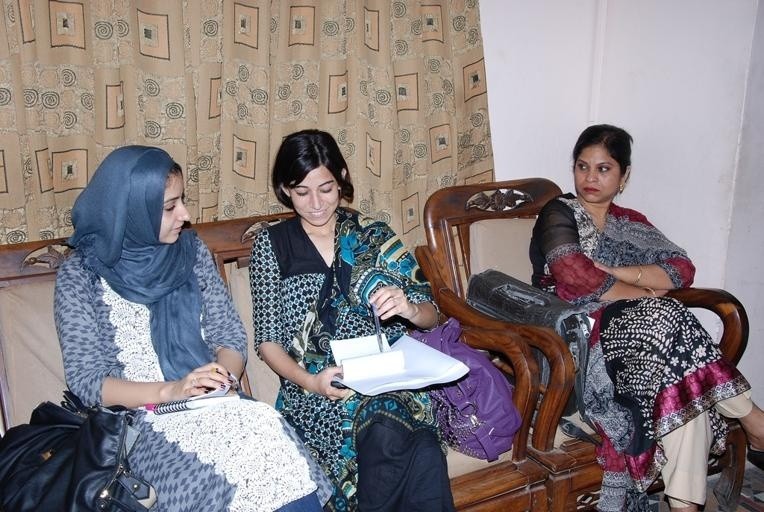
[{"x1": 415, "y1": 178, "x2": 749, "y2": 512}]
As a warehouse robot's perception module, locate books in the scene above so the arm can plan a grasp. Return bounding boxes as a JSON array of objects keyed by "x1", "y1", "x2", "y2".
[
  {"x1": 152, "y1": 374, "x2": 241, "y2": 415},
  {"x1": 329, "y1": 335, "x2": 475, "y2": 397}
]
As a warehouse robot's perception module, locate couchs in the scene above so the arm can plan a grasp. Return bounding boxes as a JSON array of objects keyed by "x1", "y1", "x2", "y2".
[{"x1": 0, "y1": 210, "x2": 546, "y2": 512}]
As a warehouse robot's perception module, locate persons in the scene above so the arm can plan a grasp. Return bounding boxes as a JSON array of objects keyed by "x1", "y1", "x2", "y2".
[
  {"x1": 251, "y1": 130, "x2": 460, "y2": 512},
  {"x1": 55, "y1": 144, "x2": 335, "y2": 511},
  {"x1": 527, "y1": 122, "x2": 764, "y2": 512}
]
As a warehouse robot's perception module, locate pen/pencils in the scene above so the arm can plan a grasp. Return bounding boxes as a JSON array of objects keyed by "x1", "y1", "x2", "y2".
[{"x1": 372, "y1": 302, "x2": 383, "y2": 353}]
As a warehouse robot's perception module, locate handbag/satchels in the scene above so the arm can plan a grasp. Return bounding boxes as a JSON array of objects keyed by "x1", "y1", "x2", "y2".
[
  {"x1": 0, "y1": 401, "x2": 127, "y2": 512},
  {"x1": 409, "y1": 318, "x2": 523, "y2": 461},
  {"x1": 467, "y1": 267, "x2": 590, "y2": 417}
]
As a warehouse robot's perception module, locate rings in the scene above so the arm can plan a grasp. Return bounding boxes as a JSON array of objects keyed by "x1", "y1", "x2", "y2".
[{"x1": 192, "y1": 377, "x2": 202, "y2": 387}]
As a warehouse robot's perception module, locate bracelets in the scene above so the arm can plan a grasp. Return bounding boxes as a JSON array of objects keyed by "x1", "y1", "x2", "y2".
[
  {"x1": 632, "y1": 264, "x2": 643, "y2": 289},
  {"x1": 643, "y1": 286, "x2": 659, "y2": 302}
]
